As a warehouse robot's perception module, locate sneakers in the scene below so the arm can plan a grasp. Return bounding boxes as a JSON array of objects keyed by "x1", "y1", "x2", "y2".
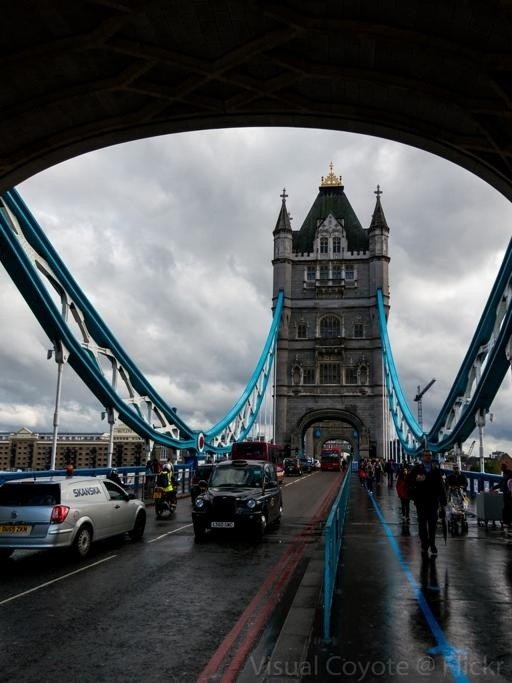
[
  {"x1": 403, "y1": 512, "x2": 409, "y2": 518},
  {"x1": 422, "y1": 545, "x2": 437, "y2": 553}
]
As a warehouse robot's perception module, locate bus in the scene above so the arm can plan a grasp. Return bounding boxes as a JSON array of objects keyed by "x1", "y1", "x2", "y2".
[{"x1": 231, "y1": 442, "x2": 285, "y2": 481}]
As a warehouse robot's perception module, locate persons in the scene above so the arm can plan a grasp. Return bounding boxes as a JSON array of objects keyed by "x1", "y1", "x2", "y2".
[
  {"x1": 490, "y1": 463, "x2": 512, "y2": 525},
  {"x1": 109, "y1": 452, "x2": 214, "y2": 515},
  {"x1": 358, "y1": 453, "x2": 469, "y2": 554},
  {"x1": 341, "y1": 459, "x2": 348, "y2": 472}
]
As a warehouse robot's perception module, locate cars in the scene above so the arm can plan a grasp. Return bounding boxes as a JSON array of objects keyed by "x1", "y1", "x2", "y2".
[
  {"x1": 190, "y1": 459, "x2": 283, "y2": 544},
  {"x1": 283, "y1": 455, "x2": 321, "y2": 476},
  {"x1": 0, "y1": 474, "x2": 147, "y2": 559}
]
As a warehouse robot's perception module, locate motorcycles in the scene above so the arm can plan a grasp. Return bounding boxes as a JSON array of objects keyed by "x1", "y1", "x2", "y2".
[{"x1": 153, "y1": 477, "x2": 181, "y2": 521}]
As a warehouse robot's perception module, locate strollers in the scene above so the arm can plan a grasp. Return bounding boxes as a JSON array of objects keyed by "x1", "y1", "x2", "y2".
[{"x1": 448, "y1": 485, "x2": 469, "y2": 537}]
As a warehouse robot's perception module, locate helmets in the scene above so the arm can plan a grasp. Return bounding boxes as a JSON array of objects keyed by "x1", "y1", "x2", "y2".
[
  {"x1": 163, "y1": 464, "x2": 171, "y2": 470},
  {"x1": 111, "y1": 468, "x2": 117, "y2": 473}
]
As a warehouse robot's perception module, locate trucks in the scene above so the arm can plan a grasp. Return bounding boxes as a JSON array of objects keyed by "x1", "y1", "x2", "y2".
[{"x1": 321, "y1": 447, "x2": 341, "y2": 471}]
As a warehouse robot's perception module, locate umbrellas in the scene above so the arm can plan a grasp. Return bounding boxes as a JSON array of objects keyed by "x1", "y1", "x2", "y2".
[{"x1": 495, "y1": 453, "x2": 512, "y2": 473}]
